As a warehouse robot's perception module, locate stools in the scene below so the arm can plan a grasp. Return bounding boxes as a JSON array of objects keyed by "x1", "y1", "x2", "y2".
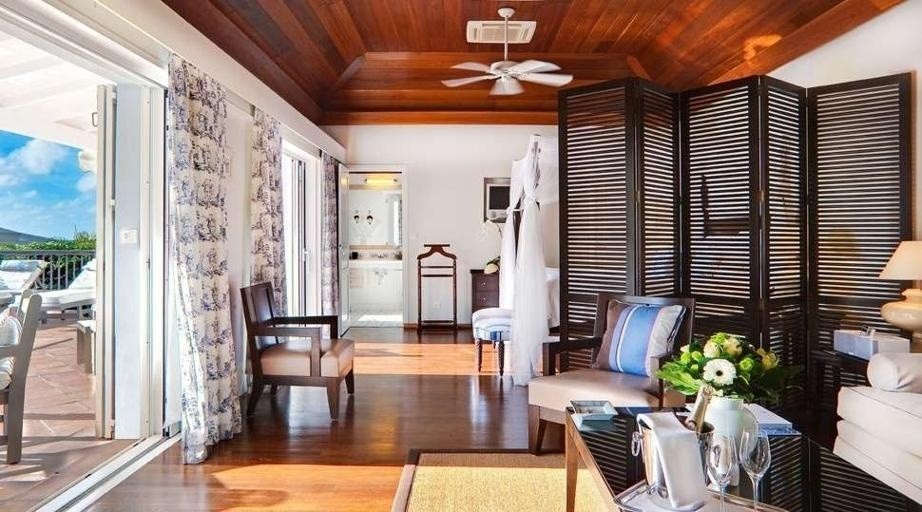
[{"x1": 471, "y1": 307, "x2": 515, "y2": 377}]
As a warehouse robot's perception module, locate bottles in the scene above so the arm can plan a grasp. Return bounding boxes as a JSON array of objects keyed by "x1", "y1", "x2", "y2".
[{"x1": 684, "y1": 386, "x2": 712, "y2": 434}]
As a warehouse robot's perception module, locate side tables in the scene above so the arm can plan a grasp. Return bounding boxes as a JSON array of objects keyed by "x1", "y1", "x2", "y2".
[{"x1": 805, "y1": 350, "x2": 870, "y2": 439}]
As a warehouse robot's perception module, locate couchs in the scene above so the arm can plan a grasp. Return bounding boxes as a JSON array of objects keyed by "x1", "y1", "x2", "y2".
[{"x1": 832, "y1": 353, "x2": 921, "y2": 511}]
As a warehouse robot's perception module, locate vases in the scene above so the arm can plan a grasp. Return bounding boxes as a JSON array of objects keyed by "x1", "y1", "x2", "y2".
[{"x1": 701, "y1": 397, "x2": 757, "y2": 477}]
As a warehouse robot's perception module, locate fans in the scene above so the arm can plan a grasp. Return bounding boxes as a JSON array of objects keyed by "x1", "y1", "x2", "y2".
[{"x1": 442, "y1": 7, "x2": 573, "y2": 96}]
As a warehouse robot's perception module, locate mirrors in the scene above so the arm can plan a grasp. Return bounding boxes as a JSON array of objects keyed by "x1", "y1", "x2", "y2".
[{"x1": 348, "y1": 184, "x2": 401, "y2": 250}]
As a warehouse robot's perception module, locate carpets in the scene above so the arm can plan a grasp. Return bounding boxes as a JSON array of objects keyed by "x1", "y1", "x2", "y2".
[{"x1": 389, "y1": 448, "x2": 614, "y2": 512}]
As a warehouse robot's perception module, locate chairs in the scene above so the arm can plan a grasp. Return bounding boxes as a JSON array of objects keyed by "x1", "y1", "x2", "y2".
[
  {"x1": 527, "y1": 291, "x2": 696, "y2": 456},
  {"x1": 0, "y1": 290, "x2": 41, "y2": 463},
  {"x1": 242, "y1": 282, "x2": 356, "y2": 421},
  {"x1": 14, "y1": 259, "x2": 97, "y2": 325},
  {"x1": 76, "y1": 304, "x2": 98, "y2": 373},
  {"x1": 1, "y1": 259, "x2": 50, "y2": 310}
]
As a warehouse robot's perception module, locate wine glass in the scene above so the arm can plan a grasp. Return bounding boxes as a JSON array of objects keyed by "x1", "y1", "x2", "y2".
[
  {"x1": 738, "y1": 430, "x2": 771, "y2": 512},
  {"x1": 706, "y1": 434, "x2": 738, "y2": 512}
]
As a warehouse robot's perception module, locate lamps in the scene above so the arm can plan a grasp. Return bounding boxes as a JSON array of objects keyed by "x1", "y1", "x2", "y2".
[
  {"x1": 878, "y1": 240, "x2": 922, "y2": 343},
  {"x1": 492, "y1": 75, "x2": 524, "y2": 97}
]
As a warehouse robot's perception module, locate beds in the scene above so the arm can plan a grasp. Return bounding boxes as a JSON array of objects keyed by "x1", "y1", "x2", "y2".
[{"x1": 498, "y1": 133, "x2": 560, "y2": 386}]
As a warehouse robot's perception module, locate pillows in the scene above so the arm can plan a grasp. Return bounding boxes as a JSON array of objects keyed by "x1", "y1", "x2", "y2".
[
  {"x1": 591, "y1": 300, "x2": 686, "y2": 378},
  {"x1": 1, "y1": 306, "x2": 22, "y2": 392}
]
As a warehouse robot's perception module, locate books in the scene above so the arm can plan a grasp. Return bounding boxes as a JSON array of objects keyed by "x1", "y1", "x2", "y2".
[{"x1": 686, "y1": 402, "x2": 793, "y2": 436}]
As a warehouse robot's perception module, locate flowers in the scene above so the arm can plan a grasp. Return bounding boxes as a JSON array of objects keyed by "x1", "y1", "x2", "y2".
[{"x1": 663, "y1": 335, "x2": 810, "y2": 408}]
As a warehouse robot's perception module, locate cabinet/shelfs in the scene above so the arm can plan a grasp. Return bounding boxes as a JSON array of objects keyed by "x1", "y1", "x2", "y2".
[
  {"x1": 349, "y1": 258, "x2": 403, "y2": 313},
  {"x1": 471, "y1": 269, "x2": 499, "y2": 313}
]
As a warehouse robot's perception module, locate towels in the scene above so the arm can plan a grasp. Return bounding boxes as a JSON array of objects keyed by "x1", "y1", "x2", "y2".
[{"x1": 634, "y1": 411, "x2": 707, "y2": 511}]
